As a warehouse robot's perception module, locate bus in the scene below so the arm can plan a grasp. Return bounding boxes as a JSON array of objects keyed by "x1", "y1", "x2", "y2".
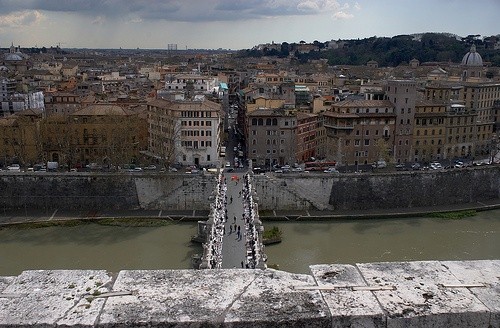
[{"x1": 305, "y1": 161, "x2": 337, "y2": 170}]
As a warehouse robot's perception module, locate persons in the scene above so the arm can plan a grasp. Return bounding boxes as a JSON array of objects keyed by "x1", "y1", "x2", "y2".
[{"x1": 208, "y1": 142, "x2": 255, "y2": 269}]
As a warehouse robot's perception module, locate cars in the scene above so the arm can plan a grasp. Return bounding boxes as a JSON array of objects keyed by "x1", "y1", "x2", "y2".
[
  {"x1": 373, "y1": 157, "x2": 489, "y2": 171},
  {"x1": 219, "y1": 104, "x2": 244, "y2": 169},
  {"x1": 6, "y1": 160, "x2": 217, "y2": 173},
  {"x1": 255, "y1": 161, "x2": 302, "y2": 173}
]
